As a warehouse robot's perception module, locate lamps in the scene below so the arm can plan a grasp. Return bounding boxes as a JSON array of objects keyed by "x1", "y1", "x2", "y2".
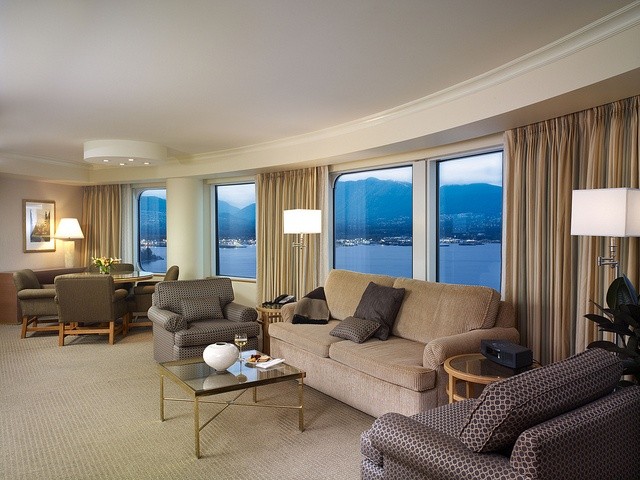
[
  {"x1": 50, "y1": 215, "x2": 86, "y2": 269},
  {"x1": 569, "y1": 187, "x2": 637, "y2": 344},
  {"x1": 280, "y1": 207, "x2": 324, "y2": 305}
]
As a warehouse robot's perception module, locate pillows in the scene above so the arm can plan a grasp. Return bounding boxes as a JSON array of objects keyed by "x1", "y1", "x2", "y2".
[
  {"x1": 182, "y1": 295, "x2": 223, "y2": 322},
  {"x1": 332, "y1": 316, "x2": 381, "y2": 345},
  {"x1": 353, "y1": 281, "x2": 406, "y2": 342}
]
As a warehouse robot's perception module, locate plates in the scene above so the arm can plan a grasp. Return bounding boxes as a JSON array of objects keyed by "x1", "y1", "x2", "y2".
[{"x1": 244, "y1": 357, "x2": 267, "y2": 364}]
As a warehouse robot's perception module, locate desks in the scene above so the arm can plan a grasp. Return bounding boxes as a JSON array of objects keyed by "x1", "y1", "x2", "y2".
[
  {"x1": 442, "y1": 350, "x2": 544, "y2": 404},
  {"x1": 74, "y1": 272, "x2": 153, "y2": 330},
  {"x1": 256, "y1": 298, "x2": 296, "y2": 356}
]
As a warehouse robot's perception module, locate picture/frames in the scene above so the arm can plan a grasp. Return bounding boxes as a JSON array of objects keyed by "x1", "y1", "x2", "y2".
[{"x1": 20, "y1": 198, "x2": 58, "y2": 254}]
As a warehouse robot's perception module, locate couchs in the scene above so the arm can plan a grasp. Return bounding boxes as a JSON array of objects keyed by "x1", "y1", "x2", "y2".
[
  {"x1": 146, "y1": 275, "x2": 264, "y2": 384},
  {"x1": 358, "y1": 344, "x2": 638, "y2": 479},
  {"x1": 52, "y1": 272, "x2": 132, "y2": 348},
  {"x1": 11, "y1": 268, "x2": 78, "y2": 339},
  {"x1": 90, "y1": 264, "x2": 136, "y2": 323},
  {"x1": 127, "y1": 264, "x2": 180, "y2": 328},
  {"x1": 268, "y1": 267, "x2": 523, "y2": 423}
]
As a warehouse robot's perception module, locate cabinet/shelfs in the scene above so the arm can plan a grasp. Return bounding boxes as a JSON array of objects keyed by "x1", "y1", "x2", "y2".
[{"x1": 0, "y1": 267, "x2": 82, "y2": 325}]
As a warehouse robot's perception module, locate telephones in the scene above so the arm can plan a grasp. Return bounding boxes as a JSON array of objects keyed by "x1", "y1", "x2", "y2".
[{"x1": 262, "y1": 294, "x2": 295, "y2": 306}]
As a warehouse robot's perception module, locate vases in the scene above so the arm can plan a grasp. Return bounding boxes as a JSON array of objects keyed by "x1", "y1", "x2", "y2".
[{"x1": 99, "y1": 265, "x2": 110, "y2": 274}]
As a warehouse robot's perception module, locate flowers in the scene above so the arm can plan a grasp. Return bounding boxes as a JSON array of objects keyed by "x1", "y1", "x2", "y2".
[{"x1": 90, "y1": 255, "x2": 121, "y2": 275}]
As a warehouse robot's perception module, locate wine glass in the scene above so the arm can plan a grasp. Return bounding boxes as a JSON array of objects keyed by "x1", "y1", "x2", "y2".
[{"x1": 235, "y1": 333, "x2": 247, "y2": 360}]
